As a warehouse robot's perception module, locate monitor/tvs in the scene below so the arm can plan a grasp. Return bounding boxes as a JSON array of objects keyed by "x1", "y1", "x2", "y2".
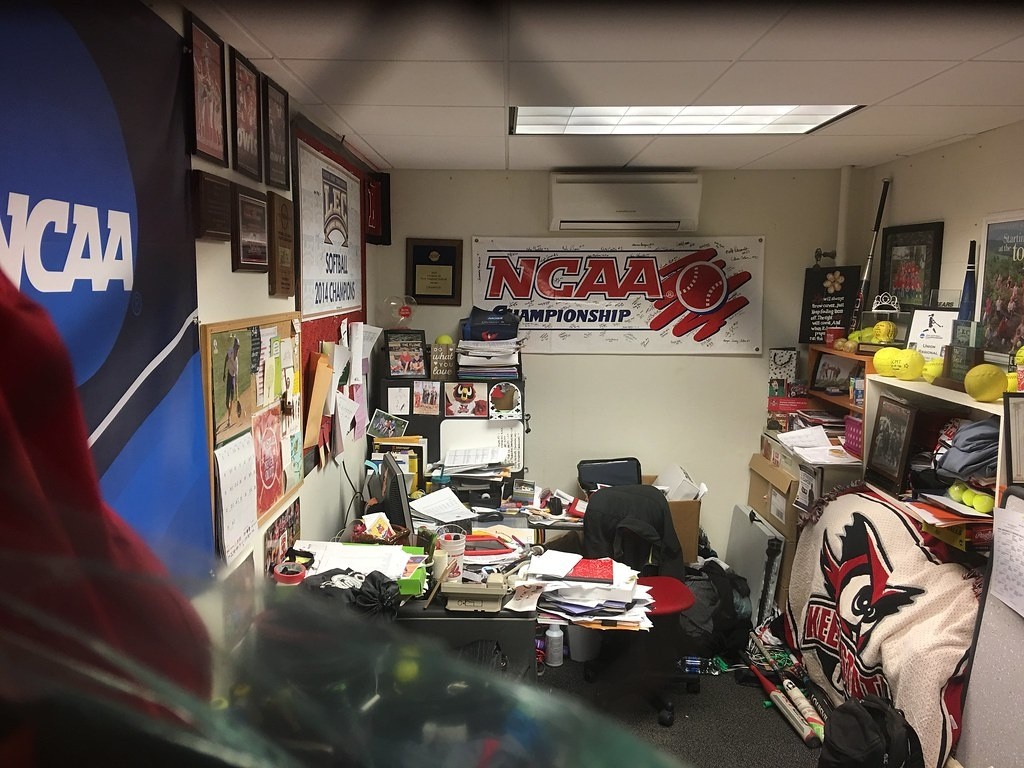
[{"x1": 354, "y1": 451, "x2": 414, "y2": 534}]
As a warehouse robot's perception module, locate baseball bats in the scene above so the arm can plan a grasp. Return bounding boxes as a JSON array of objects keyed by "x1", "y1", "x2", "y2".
[
  {"x1": 735, "y1": 627, "x2": 833, "y2": 748},
  {"x1": 847, "y1": 177, "x2": 892, "y2": 337}
]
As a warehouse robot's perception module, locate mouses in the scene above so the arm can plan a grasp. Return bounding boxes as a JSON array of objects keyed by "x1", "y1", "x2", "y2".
[{"x1": 477, "y1": 513, "x2": 504, "y2": 522}]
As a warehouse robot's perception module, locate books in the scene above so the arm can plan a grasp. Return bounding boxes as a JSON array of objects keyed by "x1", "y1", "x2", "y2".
[{"x1": 542, "y1": 559, "x2": 613, "y2": 583}]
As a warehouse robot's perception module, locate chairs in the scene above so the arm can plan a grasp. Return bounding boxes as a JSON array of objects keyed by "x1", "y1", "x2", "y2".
[{"x1": 582, "y1": 483, "x2": 700, "y2": 725}]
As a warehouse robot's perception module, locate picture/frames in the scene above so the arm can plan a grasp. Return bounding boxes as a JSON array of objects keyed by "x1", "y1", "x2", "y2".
[
  {"x1": 972, "y1": 208, "x2": 1024, "y2": 364},
  {"x1": 383, "y1": 328, "x2": 429, "y2": 379},
  {"x1": 810, "y1": 351, "x2": 864, "y2": 392},
  {"x1": 183, "y1": 9, "x2": 295, "y2": 299},
  {"x1": 864, "y1": 396, "x2": 915, "y2": 500},
  {"x1": 879, "y1": 221, "x2": 944, "y2": 311}
]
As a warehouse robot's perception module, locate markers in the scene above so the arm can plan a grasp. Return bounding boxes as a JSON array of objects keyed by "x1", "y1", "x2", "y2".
[{"x1": 498, "y1": 507, "x2": 525, "y2": 511}]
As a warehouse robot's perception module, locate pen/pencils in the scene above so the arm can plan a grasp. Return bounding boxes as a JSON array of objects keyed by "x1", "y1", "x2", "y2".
[{"x1": 512, "y1": 535, "x2": 525, "y2": 547}]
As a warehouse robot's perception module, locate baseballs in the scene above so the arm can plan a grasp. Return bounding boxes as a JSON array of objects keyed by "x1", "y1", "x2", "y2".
[{"x1": 948, "y1": 483, "x2": 995, "y2": 512}]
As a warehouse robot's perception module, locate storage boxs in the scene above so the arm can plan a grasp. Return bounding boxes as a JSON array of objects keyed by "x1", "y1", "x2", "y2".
[
  {"x1": 768, "y1": 395, "x2": 807, "y2": 431},
  {"x1": 275, "y1": 540, "x2": 427, "y2": 596},
  {"x1": 577, "y1": 461, "x2": 701, "y2": 564},
  {"x1": 747, "y1": 453, "x2": 799, "y2": 604},
  {"x1": 922, "y1": 520, "x2": 993, "y2": 553}
]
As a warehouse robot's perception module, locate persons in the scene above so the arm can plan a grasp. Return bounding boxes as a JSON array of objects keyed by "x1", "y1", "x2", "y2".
[
  {"x1": 223, "y1": 338, "x2": 240, "y2": 426},
  {"x1": 893, "y1": 260, "x2": 922, "y2": 298},
  {"x1": 983, "y1": 274, "x2": 1024, "y2": 351},
  {"x1": 375, "y1": 416, "x2": 396, "y2": 437},
  {"x1": 415, "y1": 382, "x2": 437, "y2": 407},
  {"x1": 398, "y1": 349, "x2": 424, "y2": 372},
  {"x1": 272, "y1": 502, "x2": 300, "y2": 542}
]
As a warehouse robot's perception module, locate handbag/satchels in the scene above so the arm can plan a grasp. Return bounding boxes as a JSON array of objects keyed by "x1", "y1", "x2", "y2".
[
  {"x1": 298, "y1": 568, "x2": 402, "y2": 640},
  {"x1": 468, "y1": 306, "x2": 519, "y2": 339}
]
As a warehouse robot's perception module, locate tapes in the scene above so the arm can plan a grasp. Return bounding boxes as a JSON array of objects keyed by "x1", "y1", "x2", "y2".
[
  {"x1": 274, "y1": 562, "x2": 307, "y2": 584},
  {"x1": 482, "y1": 493, "x2": 491, "y2": 499}
]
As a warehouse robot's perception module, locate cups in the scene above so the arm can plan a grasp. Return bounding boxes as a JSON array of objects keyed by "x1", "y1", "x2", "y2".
[
  {"x1": 433, "y1": 550, "x2": 448, "y2": 579},
  {"x1": 439, "y1": 532, "x2": 465, "y2": 583}
]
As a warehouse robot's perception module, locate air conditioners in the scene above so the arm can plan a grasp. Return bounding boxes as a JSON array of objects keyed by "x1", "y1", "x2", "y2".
[{"x1": 547, "y1": 170, "x2": 701, "y2": 233}]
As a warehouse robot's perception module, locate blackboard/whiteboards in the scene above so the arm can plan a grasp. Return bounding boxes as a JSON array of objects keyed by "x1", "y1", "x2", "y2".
[{"x1": 799, "y1": 266, "x2": 862, "y2": 344}]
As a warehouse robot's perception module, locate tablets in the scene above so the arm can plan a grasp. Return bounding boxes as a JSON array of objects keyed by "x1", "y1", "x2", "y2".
[{"x1": 464, "y1": 538, "x2": 512, "y2": 556}]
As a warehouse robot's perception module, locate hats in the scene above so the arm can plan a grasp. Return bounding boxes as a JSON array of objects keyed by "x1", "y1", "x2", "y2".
[{"x1": 234, "y1": 339, "x2": 240, "y2": 347}]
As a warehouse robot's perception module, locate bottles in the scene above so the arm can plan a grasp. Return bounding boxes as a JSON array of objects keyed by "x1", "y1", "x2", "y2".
[
  {"x1": 545, "y1": 625, "x2": 565, "y2": 667},
  {"x1": 677, "y1": 656, "x2": 721, "y2": 675}
]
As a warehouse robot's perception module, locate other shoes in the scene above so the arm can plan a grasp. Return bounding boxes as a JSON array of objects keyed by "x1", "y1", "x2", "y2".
[{"x1": 228, "y1": 417, "x2": 230, "y2": 426}]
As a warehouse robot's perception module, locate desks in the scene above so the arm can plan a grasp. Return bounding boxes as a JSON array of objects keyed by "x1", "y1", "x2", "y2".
[{"x1": 398, "y1": 513, "x2": 582, "y2": 686}]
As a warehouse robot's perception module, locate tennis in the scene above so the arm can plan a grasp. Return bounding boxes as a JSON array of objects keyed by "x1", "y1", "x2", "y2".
[
  {"x1": 435, "y1": 335, "x2": 453, "y2": 344},
  {"x1": 848, "y1": 321, "x2": 1024, "y2": 402}
]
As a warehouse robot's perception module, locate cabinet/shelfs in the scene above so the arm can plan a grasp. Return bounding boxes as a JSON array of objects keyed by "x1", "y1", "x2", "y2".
[
  {"x1": 759, "y1": 428, "x2": 863, "y2": 498},
  {"x1": 863, "y1": 372, "x2": 1007, "y2": 558},
  {"x1": 804, "y1": 342, "x2": 876, "y2": 418}
]
